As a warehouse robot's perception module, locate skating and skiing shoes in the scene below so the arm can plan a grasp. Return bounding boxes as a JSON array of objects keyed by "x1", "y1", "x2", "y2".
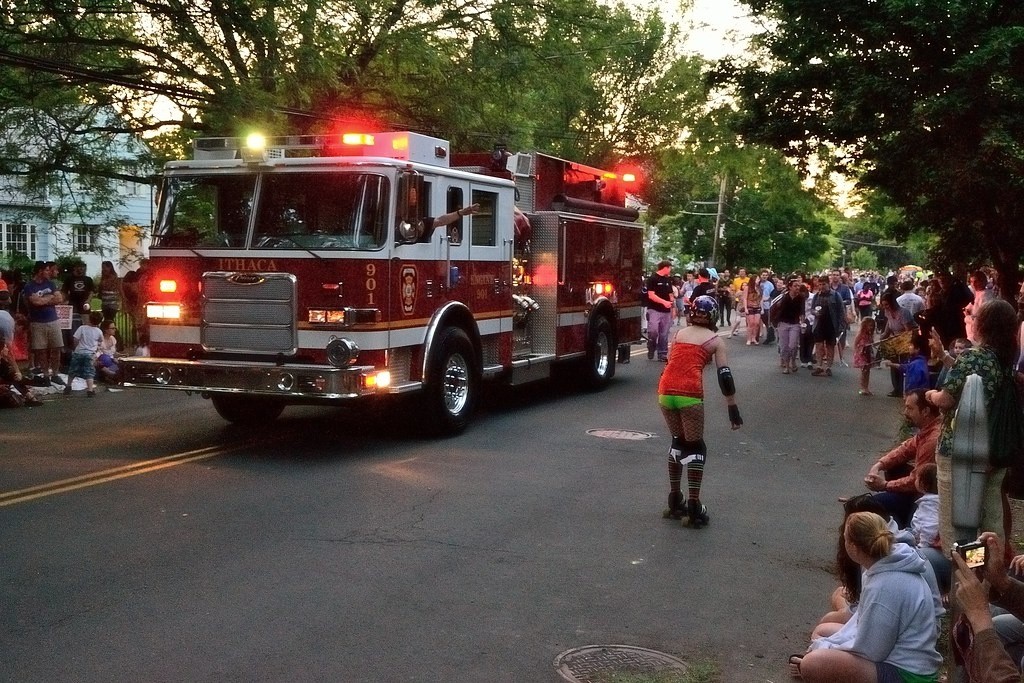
[{"x1": 663, "y1": 489, "x2": 709, "y2": 530}]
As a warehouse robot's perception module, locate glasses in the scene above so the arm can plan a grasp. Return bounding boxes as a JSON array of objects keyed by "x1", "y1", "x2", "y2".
[
  {"x1": 843, "y1": 493, "x2": 873, "y2": 514},
  {"x1": 971, "y1": 314, "x2": 977, "y2": 320},
  {"x1": 109, "y1": 327, "x2": 117, "y2": 330}
]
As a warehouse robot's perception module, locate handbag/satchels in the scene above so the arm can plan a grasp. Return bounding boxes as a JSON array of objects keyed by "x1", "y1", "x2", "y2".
[
  {"x1": 844, "y1": 304, "x2": 857, "y2": 324},
  {"x1": 737, "y1": 286, "x2": 748, "y2": 312}
]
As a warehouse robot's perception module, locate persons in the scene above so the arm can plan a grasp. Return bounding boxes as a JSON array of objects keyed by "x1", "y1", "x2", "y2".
[
  {"x1": 908, "y1": 463, "x2": 961, "y2": 586},
  {"x1": 852, "y1": 268, "x2": 918, "y2": 335},
  {"x1": 657, "y1": 295, "x2": 744, "y2": 529},
  {"x1": 646, "y1": 262, "x2": 677, "y2": 362},
  {"x1": 810, "y1": 493, "x2": 946, "y2": 644},
  {"x1": 642, "y1": 268, "x2": 858, "y2": 366},
  {"x1": 1, "y1": 259, "x2": 151, "y2": 409},
  {"x1": 810, "y1": 275, "x2": 848, "y2": 376},
  {"x1": 951, "y1": 533, "x2": 1024, "y2": 683},
  {"x1": 924, "y1": 300, "x2": 1021, "y2": 569},
  {"x1": 916, "y1": 264, "x2": 1024, "y2": 566},
  {"x1": 393, "y1": 202, "x2": 480, "y2": 242},
  {"x1": 866, "y1": 388, "x2": 945, "y2": 526},
  {"x1": 885, "y1": 335, "x2": 931, "y2": 396},
  {"x1": 881, "y1": 292, "x2": 920, "y2": 399},
  {"x1": 853, "y1": 317, "x2": 877, "y2": 396},
  {"x1": 770, "y1": 279, "x2": 807, "y2": 374},
  {"x1": 788, "y1": 511, "x2": 943, "y2": 683}
]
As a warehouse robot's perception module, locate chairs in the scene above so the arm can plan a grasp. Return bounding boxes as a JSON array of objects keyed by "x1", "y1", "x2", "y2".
[{"x1": 864, "y1": 329, "x2": 914, "y2": 366}]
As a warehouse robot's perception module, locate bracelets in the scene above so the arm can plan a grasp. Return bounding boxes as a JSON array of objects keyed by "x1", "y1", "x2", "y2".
[
  {"x1": 937, "y1": 349, "x2": 949, "y2": 361},
  {"x1": 457, "y1": 209, "x2": 462, "y2": 216}
]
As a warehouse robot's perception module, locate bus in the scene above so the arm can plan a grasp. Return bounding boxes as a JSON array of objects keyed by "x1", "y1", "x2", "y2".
[{"x1": 898, "y1": 264, "x2": 922, "y2": 279}]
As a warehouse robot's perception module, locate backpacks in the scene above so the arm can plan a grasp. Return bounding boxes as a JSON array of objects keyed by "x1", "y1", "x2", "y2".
[{"x1": 975, "y1": 343, "x2": 1024, "y2": 468}]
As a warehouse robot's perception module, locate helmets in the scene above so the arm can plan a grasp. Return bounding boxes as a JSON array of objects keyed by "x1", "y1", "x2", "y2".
[{"x1": 689, "y1": 295, "x2": 721, "y2": 332}]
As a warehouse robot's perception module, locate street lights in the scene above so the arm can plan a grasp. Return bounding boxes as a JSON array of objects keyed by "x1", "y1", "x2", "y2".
[{"x1": 842, "y1": 250, "x2": 846, "y2": 267}]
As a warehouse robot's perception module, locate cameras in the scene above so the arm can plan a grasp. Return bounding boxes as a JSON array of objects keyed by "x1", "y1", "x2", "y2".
[{"x1": 956, "y1": 540, "x2": 988, "y2": 569}]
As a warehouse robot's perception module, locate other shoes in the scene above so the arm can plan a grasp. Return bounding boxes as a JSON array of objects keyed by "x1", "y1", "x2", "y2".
[
  {"x1": 26, "y1": 396, "x2": 42, "y2": 406},
  {"x1": 887, "y1": 391, "x2": 903, "y2": 397},
  {"x1": 86, "y1": 390, "x2": 96, "y2": 397},
  {"x1": 763, "y1": 335, "x2": 776, "y2": 345},
  {"x1": 42, "y1": 377, "x2": 51, "y2": 386},
  {"x1": 648, "y1": 348, "x2": 656, "y2": 359},
  {"x1": 825, "y1": 368, "x2": 832, "y2": 376},
  {"x1": 52, "y1": 375, "x2": 63, "y2": 385},
  {"x1": 792, "y1": 365, "x2": 798, "y2": 372},
  {"x1": 733, "y1": 328, "x2": 740, "y2": 336},
  {"x1": 657, "y1": 357, "x2": 668, "y2": 362},
  {"x1": 811, "y1": 368, "x2": 824, "y2": 376},
  {"x1": 728, "y1": 321, "x2": 731, "y2": 326},
  {"x1": 874, "y1": 363, "x2": 881, "y2": 369},
  {"x1": 63, "y1": 386, "x2": 72, "y2": 395},
  {"x1": 782, "y1": 368, "x2": 789, "y2": 374},
  {"x1": 858, "y1": 388, "x2": 872, "y2": 396},
  {"x1": 799, "y1": 362, "x2": 813, "y2": 370},
  {"x1": 719, "y1": 324, "x2": 724, "y2": 327},
  {"x1": 727, "y1": 334, "x2": 733, "y2": 339}
]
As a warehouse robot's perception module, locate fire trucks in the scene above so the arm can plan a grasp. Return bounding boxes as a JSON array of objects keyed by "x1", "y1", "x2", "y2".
[{"x1": 115, "y1": 131, "x2": 644, "y2": 434}]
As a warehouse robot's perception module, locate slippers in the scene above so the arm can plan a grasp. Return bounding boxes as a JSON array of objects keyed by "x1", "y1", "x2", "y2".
[{"x1": 789, "y1": 654, "x2": 804, "y2": 677}]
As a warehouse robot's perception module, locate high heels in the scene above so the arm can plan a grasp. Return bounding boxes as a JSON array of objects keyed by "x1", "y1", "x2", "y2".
[
  {"x1": 745, "y1": 338, "x2": 752, "y2": 346},
  {"x1": 754, "y1": 335, "x2": 760, "y2": 345}
]
